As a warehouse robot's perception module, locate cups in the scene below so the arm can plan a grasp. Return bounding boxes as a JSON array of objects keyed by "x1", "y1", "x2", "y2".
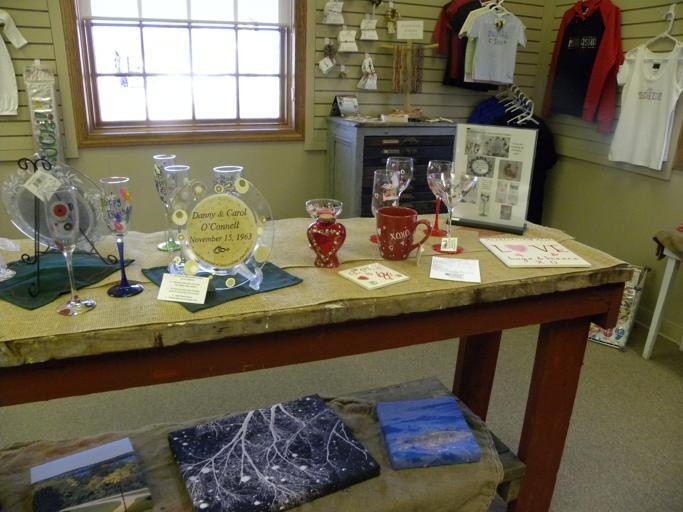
[
  {"x1": 305, "y1": 198, "x2": 344, "y2": 222},
  {"x1": 376, "y1": 206, "x2": 432, "y2": 261}
]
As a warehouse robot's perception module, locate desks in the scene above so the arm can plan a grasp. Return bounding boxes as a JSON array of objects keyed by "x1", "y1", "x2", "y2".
[{"x1": 0, "y1": 213, "x2": 630, "y2": 512}]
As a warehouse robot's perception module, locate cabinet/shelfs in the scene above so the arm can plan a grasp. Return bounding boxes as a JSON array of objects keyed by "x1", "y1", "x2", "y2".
[{"x1": 325, "y1": 116, "x2": 457, "y2": 218}]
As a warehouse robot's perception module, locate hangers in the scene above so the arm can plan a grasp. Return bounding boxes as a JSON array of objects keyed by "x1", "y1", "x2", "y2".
[
  {"x1": 625, "y1": 9, "x2": 683, "y2": 61},
  {"x1": 480, "y1": 0, "x2": 510, "y2": 14},
  {"x1": 497, "y1": 83, "x2": 540, "y2": 126}
]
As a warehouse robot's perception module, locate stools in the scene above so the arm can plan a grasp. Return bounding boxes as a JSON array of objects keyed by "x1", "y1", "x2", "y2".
[{"x1": 642, "y1": 230, "x2": 683, "y2": 360}]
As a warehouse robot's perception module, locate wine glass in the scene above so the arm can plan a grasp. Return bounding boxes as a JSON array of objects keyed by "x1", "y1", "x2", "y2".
[
  {"x1": 427, "y1": 160, "x2": 457, "y2": 237},
  {"x1": 43, "y1": 185, "x2": 96, "y2": 315},
  {"x1": 370, "y1": 156, "x2": 414, "y2": 246},
  {"x1": 212, "y1": 165, "x2": 244, "y2": 197},
  {"x1": 426, "y1": 172, "x2": 479, "y2": 255},
  {"x1": 99, "y1": 176, "x2": 144, "y2": 298},
  {"x1": 151, "y1": 153, "x2": 192, "y2": 275}
]
